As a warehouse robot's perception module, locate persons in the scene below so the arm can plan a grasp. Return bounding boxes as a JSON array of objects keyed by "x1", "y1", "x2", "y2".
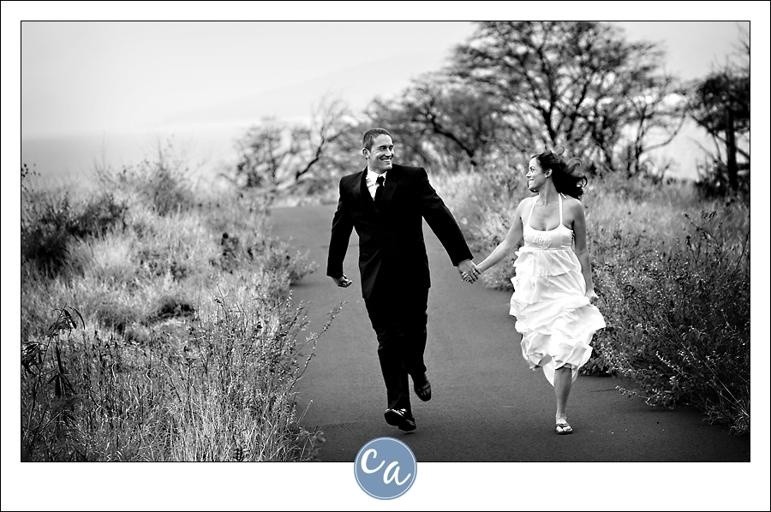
[
  {"x1": 459, "y1": 151, "x2": 609, "y2": 436},
  {"x1": 327, "y1": 127, "x2": 483, "y2": 432}
]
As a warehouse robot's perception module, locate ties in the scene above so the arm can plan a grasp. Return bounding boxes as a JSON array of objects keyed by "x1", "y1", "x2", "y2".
[{"x1": 375, "y1": 176, "x2": 385, "y2": 202}]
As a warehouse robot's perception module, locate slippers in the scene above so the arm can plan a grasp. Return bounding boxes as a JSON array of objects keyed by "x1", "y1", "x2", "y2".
[{"x1": 554, "y1": 423, "x2": 572, "y2": 435}]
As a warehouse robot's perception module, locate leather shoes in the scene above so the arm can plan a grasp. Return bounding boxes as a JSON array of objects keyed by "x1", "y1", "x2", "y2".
[
  {"x1": 414, "y1": 376, "x2": 431, "y2": 402},
  {"x1": 384, "y1": 408, "x2": 416, "y2": 432}
]
